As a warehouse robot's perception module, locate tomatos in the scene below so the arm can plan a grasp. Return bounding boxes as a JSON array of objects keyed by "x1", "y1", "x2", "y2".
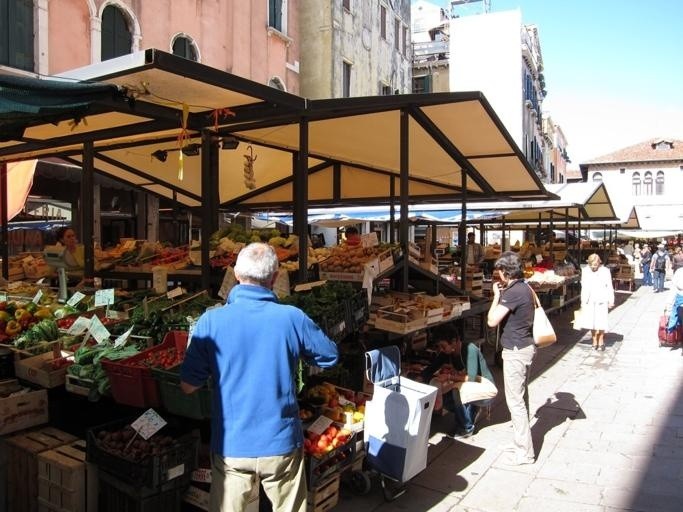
[
  {"x1": 123, "y1": 346, "x2": 185, "y2": 369},
  {"x1": 58, "y1": 316, "x2": 120, "y2": 329},
  {"x1": 303, "y1": 423, "x2": 350, "y2": 456}
]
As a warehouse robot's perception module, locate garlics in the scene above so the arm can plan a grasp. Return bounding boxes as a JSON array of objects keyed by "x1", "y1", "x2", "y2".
[{"x1": 243, "y1": 161, "x2": 255, "y2": 190}]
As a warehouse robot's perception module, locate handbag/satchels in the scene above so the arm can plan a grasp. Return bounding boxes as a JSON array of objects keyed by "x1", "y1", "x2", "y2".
[
  {"x1": 641, "y1": 251, "x2": 651, "y2": 264},
  {"x1": 525, "y1": 282, "x2": 557, "y2": 348},
  {"x1": 573, "y1": 308, "x2": 583, "y2": 331}
]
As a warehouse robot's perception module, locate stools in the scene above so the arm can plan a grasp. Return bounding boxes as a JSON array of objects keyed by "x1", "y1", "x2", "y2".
[{"x1": 468, "y1": 400, "x2": 493, "y2": 425}]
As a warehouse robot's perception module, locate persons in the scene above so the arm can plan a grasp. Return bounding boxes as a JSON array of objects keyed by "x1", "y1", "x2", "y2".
[
  {"x1": 609, "y1": 233, "x2": 682, "y2": 293},
  {"x1": 486, "y1": 249, "x2": 539, "y2": 462},
  {"x1": 576, "y1": 252, "x2": 616, "y2": 350},
  {"x1": 412, "y1": 319, "x2": 500, "y2": 441},
  {"x1": 179, "y1": 242, "x2": 342, "y2": 512},
  {"x1": 53, "y1": 224, "x2": 95, "y2": 270},
  {"x1": 343, "y1": 225, "x2": 362, "y2": 240},
  {"x1": 461, "y1": 232, "x2": 485, "y2": 273}
]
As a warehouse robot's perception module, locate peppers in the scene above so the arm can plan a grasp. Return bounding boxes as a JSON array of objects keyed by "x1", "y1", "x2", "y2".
[
  {"x1": 0, "y1": 302, "x2": 50, "y2": 347},
  {"x1": 298, "y1": 382, "x2": 367, "y2": 422}
]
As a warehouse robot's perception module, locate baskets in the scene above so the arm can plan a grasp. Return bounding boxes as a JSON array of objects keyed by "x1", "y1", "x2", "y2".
[
  {"x1": 84, "y1": 416, "x2": 198, "y2": 489},
  {"x1": 314, "y1": 300, "x2": 350, "y2": 344},
  {"x1": 346, "y1": 288, "x2": 369, "y2": 334}
]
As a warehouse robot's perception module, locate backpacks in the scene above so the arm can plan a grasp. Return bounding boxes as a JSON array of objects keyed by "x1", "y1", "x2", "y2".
[{"x1": 654, "y1": 252, "x2": 666, "y2": 270}]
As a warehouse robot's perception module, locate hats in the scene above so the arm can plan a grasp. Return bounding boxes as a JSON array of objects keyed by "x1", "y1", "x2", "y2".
[
  {"x1": 656, "y1": 244, "x2": 664, "y2": 248},
  {"x1": 642, "y1": 244, "x2": 648, "y2": 247}
]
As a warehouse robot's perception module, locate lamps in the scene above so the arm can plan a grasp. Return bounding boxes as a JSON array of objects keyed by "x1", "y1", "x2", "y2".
[{"x1": 152, "y1": 137, "x2": 238, "y2": 162}]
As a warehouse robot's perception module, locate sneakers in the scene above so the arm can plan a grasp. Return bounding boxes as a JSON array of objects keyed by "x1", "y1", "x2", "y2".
[
  {"x1": 592, "y1": 345, "x2": 598, "y2": 350},
  {"x1": 446, "y1": 424, "x2": 474, "y2": 438},
  {"x1": 501, "y1": 451, "x2": 534, "y2": 465},
  {"x1": 597, "y1": 344, "x2": 605, "y2": 351}
]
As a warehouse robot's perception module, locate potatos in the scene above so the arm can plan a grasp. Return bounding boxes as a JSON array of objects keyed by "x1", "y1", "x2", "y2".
[
  {"x1": 100, "y1": 428, "x2": 173, "y2": 456},
  {"x1": 322, "y1": 244, "x2": 385, "y2": 273}
]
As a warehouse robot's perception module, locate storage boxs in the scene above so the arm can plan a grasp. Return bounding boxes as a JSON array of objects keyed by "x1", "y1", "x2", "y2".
[
  {"x1": 100, "y1": 331, "x2": 187, "y2": 408},
  {"x1": 151, "y1": 361, "x2": 212, "y2": 421},
  {"x1": 551, "y1": 295, "x2": 564, "y2": 307},
  {"x1": 319, "y1": 246, "x2": 396, "y2": 281},
  {"x1": 183, "y1": 381, "x2": 373, "y2": 512},
  {"x1": 552, "y1": 285, "x2": 567, "y2": 295},
  {"x1": 0, "y1": 353, "x2": 99, "y2": 512},
  {"x1": 407, "y1": 241, "x2": 439, "y2": 275},
  {"x1": 0, "y1": 240, "x2": 216, "y2": 353},
  {"x1": 465, "y1": 266, "x2": 483, "y2": 299},
  {"x1": 365, "y1": 290, "x2": 473, "y2": 336}
]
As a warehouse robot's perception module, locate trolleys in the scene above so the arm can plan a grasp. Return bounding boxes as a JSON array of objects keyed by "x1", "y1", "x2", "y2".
[
  {"x1": 658, "y1": 306, "x2": 683, "y2": 349},
  {"x1": 347, "y1": 345, "x2": 438, "y2": 500}
]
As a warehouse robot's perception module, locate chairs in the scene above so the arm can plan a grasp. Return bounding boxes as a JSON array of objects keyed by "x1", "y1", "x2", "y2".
[{"x1": 613, "y1": 264, "x2": 636, "y2": 295}]
